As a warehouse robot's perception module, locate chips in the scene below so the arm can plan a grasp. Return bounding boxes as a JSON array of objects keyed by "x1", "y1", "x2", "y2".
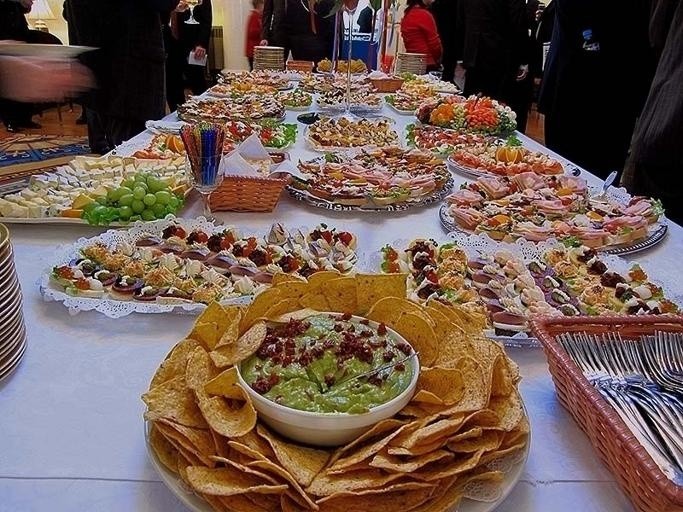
[{"x1": 139, "y1": 271, "x2": 530, "y2": 511}]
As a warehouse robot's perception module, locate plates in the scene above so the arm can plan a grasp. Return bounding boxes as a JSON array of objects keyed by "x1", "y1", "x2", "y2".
[
  {"x1": 207, "y1": 84, "x2": 280, "y2": 101},
  {"x1": 382, "y1": 92, "x2": 467, "y2": 115},
  {"x1": 303, "y1": 124, "x2": 403, "y2": 153},
  {"x1": 276, "y1": 92, "x2": 313, "y2": 112},
  {"x1": 414, "y1": 130, "x2": 509, "y2": 155},
  {"x1": 144, "y1": 119, "x2": 189, "y2": 138},
  {"x1": 315, "y1": 95, "x2": 383, "y2": 114},
  {"x1": 40, "y1": 222, "x2": 359, "y2": 317},
  {"x1": 0, "y1": 40, "x2": 101, "y2": 63},
  {"x1": 447, "y1": 148, "x2": 582, "y2": 183},
  {"x1": 143, "y1": 334, "x2": 532, "y2": 511},
  {"x1": 0, "y1": 223, "x2": 31, "y2": 385},
  {"x1": 252, "y1": 44, "x2": 286, "y2": 73},
  {"x1": 284, "y1": 152, "x2": 454, "y2": 216},
  {"x1": 176, "y1": 100, "x2": 288, "y2": 124},
  {"x1": 437, "y1": 182, "x2": 667, "y2": 256},
  {"x1": 413, "y1": 106, "x2": 517, "y2": 136},
  {"x1": 393, "y1": 50, "x2": 427, "y2": 78}
]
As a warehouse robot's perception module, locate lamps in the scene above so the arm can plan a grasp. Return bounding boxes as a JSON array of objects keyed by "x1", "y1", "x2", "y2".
[{"x1": 25, "y1": 0, "x2": 56, "y2": 30}]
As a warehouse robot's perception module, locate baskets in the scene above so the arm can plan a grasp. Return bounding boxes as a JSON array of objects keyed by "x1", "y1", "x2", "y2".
[
  {"x1": 369, "y1": 78, "x2": 405, "y2": 93},
  {"x1": 211, "y1": 152, "x2": 288, "y2": 212},
  {"x1": 528, "y1": 314, "x2": 683, "y2": 512}
]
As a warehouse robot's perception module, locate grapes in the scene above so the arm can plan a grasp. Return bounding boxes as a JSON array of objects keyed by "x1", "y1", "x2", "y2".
[{"x1": 95, "y1": 172, "x2": 179, "y2": 220}]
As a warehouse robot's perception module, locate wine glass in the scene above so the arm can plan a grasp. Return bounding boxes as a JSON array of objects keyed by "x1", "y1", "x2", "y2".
[
  {"x1": 185, "y1": 153, "x2": 224, "y2": 226},
  {"x1": 183, "y1": 0, "x2": 202, "y2": 25}
]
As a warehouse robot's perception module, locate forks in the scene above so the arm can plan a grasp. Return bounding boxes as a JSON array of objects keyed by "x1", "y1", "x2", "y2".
[{"x1": 554, "y1": 326, "x2": 683, "y2": 491}]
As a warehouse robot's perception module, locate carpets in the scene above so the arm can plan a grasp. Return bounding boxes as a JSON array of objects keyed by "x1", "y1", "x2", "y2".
[{"x1": 0, "y1": 129, "x2": 105, "y2": 197}]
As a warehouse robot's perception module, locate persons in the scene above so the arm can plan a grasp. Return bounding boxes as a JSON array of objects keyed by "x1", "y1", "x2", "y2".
[
  {"x1": 245, "y1": 0, "x2": 445, "y2": 80},
  {"x1": 423, "y1": 2, "x2": 683, "y2": 227},
  {"x1": 1, "y1": 0, "x2": 213, "y2": 156}
]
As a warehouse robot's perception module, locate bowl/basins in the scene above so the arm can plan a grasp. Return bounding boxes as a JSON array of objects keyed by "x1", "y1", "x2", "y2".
[{"x1": 232, "y1": 307, "x2": 422, "y2": 450}]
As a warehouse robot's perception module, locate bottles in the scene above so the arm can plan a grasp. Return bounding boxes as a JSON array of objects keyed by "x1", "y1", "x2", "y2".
[{"x1": 582, "y1": 28, "x2": 601, "y2": 54}]
